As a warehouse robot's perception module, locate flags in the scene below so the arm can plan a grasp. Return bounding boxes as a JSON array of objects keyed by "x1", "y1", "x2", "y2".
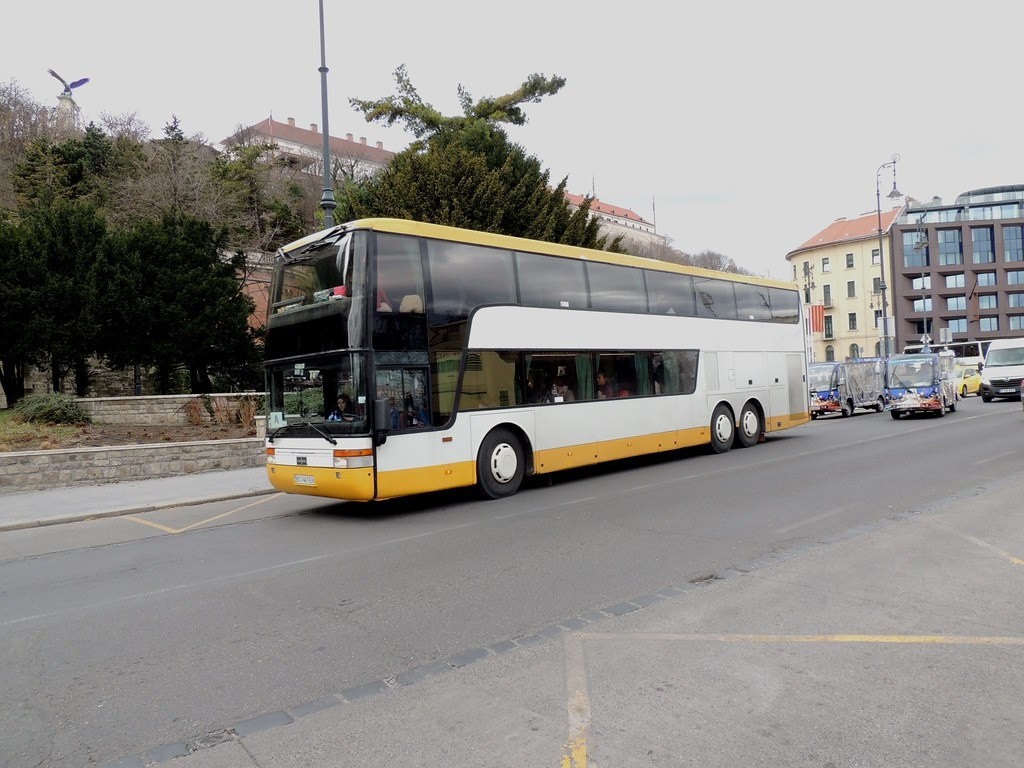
[{"x1": 809, "y1": 303, "x2": 826, "y2": 334}]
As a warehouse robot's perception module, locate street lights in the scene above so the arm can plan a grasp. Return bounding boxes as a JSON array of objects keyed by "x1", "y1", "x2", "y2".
[
  {"x1": 875, "y1": 153, "x2": 903, "y2": 420},
  {"x1": 914, "y1": 208, "x2": 933, "y2": 355}
]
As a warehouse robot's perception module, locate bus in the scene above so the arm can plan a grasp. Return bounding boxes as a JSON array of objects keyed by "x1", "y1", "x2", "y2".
[
  {"x1": 264, "y1": 216, "x2": 813, "y2": 503},
  {"x1": 901, "y1": 340, "x2": 993, "y2": 382}
]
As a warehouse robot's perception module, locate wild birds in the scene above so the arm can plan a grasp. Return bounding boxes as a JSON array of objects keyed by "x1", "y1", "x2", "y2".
[{"x1": 47, "y1": 69, "x2": 90, "y2": 95}]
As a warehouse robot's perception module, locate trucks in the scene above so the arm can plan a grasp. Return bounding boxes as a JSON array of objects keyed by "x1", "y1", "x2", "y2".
[
  {"x1": 887, "y1": 354, "x2": 959, "y2": 418},
  {"x1": 802, "y1": 358, "x2": 887, "y2": 419}
]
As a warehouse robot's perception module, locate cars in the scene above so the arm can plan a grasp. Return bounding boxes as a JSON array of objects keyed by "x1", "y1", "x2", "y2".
[{"x1": 953, "y1": 365, "x2": 984, "y2": 398}]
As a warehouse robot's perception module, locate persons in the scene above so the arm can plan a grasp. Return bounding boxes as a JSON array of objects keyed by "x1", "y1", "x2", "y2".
[
  {"x1": 595, "y1": 371, "x2": 614, "y2": 400},
  {"x1": 551, "y1": 378, "x2": 575, "y2": 402},
  {"x1": 328, "y1": 394, "x2": 360, "y2": 424},
  {"x1": 419, "y1": 401, "x2": 428, "y2": 426}
]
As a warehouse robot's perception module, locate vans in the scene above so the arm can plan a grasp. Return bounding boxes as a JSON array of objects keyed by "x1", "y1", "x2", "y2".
[{"x1": 980, "y1": 338, "x2": 1024, "y2": 403}]
